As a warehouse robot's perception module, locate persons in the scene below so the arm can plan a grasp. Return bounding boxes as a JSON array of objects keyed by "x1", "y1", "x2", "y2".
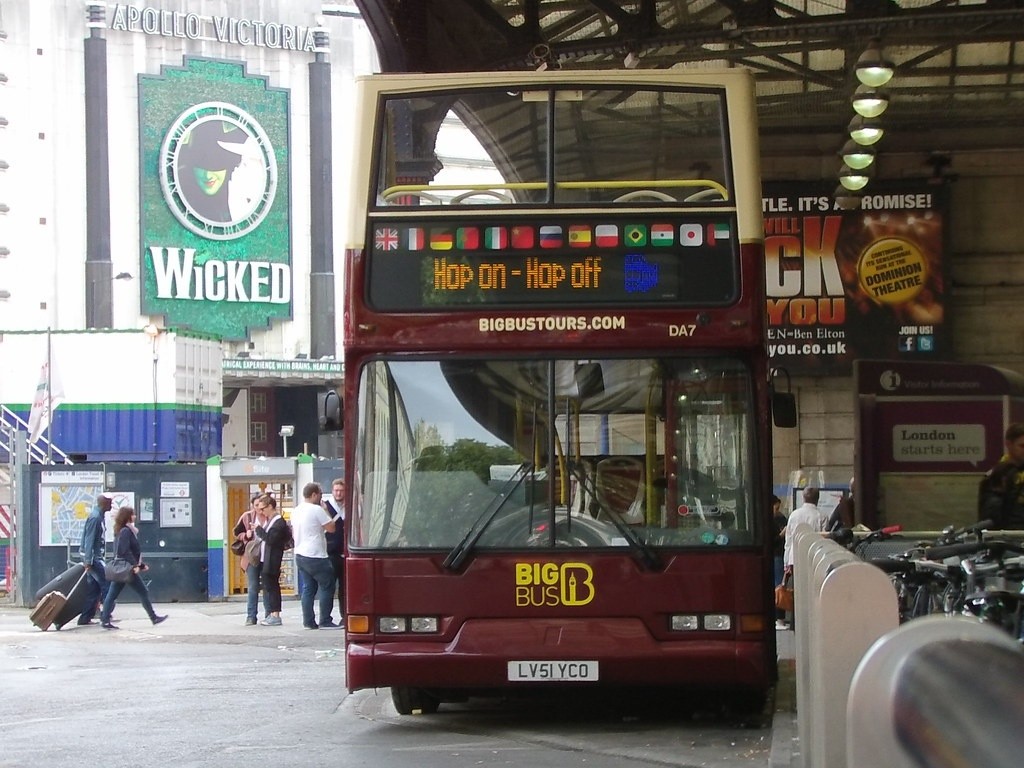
[
  {"x1": 232, "y1": 490, "x2": 286, "y2": 626},
  {"x1": 977, "y1": 422, "x2": 1024, "y2": 542},
  {"x1": 100, "y1": 506, "x2": 169, "y2": 631},
  {"x1": 290, "y1": 482, "x2": 340, "y2": 630},
  {"x1": 822, "y1": 476, "x2": 854, "y2": 532},
  {"x1": 784, "y1": 486, "x2": 829, "y2": 636},
  {"x1": 774, "y1": 495, "x2": 787, "y2": 631},
  {"x1": 324, "y1": 478, "x2": 345, "y2": 628},
  {"x1": 76, "y1": 493, "x2": 122, "y2": 625},
  {"x1": 28, "y1": 343, "x2": 66, "y2": 444}
]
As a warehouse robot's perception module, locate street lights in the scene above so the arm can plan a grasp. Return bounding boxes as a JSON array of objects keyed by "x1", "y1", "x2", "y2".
[{"x1": 279, "y1": 424, "x2": 294, "y2": 501}]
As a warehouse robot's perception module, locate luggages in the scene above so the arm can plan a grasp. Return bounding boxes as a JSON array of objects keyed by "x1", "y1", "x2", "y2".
[{"x1": 30, "y1": 562, "x2": 89, "y2": 631}]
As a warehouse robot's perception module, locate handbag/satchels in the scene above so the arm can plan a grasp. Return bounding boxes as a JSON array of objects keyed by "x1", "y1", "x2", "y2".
[
  {"x1": 104, "y1": 559, "x2": 136, "y2": 583},
  {"x1": 231, "y1": 539, "x2": 246, "y2": 557},
  {"x1": 775, "y1": 569, "x2": 794, "y2": 611},
  {"x1": 282, "y1": 528, "x2": 294, "y2": 549}
]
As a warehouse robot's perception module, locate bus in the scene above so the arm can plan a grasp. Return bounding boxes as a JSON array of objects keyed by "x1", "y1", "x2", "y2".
[{"x1": 316, "y1": 68, "x2": 798, "y2": 729}]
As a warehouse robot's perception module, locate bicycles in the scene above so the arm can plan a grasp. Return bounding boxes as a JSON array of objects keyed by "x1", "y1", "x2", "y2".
[{"x1": 820, "y1": 521, "x2": 1024, "y2": 647}]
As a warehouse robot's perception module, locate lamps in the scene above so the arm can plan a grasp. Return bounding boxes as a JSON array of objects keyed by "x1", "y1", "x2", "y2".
[
  {"x1": 295, "y1": 354, "x2": 308, "y2": 358},
  {"x1": 850, "y1": 84, "x2": 890, "y2": 117},
  {"x1": 853, "y1": 37, "x2": 897, "y2": 88},
  {"x1": 90, "y1": 272, "x2": 134, "y2": 328},
  {"x1": 841, "y1": 140, "x2": 875, "y2": 170},
  {"x1": 838, "y1": 163, "x2": 870, "y2": 190},
  {"x1": 847, "y1": 114, "x2": 884, "y2": 145},
  {"x1": 280, "y1": 425, "x2": 295, "y2": 457},
  {"x1": 236, "y1": 352, "x2": 249, "y2": 357}
]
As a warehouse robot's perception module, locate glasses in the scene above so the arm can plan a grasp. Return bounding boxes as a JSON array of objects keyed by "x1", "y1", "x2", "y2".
[{"x1": 260, "y1": 503, "x2": 270, "y2": 511}]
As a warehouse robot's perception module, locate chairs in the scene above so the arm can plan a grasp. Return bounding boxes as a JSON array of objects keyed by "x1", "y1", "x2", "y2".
[
  {"x1": 389, "y1": 189, "x2": 514, "y2": 207},
  {"x1": 612, "y1": 186, "x2": 721, "y2": 203},
  {"x1": 546, "y1": 454, "x2": 668, "y2": 521}
]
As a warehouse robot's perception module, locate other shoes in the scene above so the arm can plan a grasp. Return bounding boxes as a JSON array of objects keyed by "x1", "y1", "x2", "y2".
[
  {"x1": 304, "y1": 622, "x2": 318, "y2": 630},
  {"x1": 246, "y1": 616, "x2": 256, "y2": 625},
  {"x1": 262, "y1": 615, "x2": 282, "y2": 626},
  {"x1": 319, "y1": 621, "x2": 344, "y2": 630},
  {"x1": 153, "y1": 614, "x2": 168, "y2": 626},
  {"x1": 101, "y1": 622, "x2": 119, "y2": 629},
  {"x1": 77, "y1": 620, "x2": 99, "y2": 625},
  {"x1": 775, "y1": 620, "x2": 791, "y2": 630}
]
